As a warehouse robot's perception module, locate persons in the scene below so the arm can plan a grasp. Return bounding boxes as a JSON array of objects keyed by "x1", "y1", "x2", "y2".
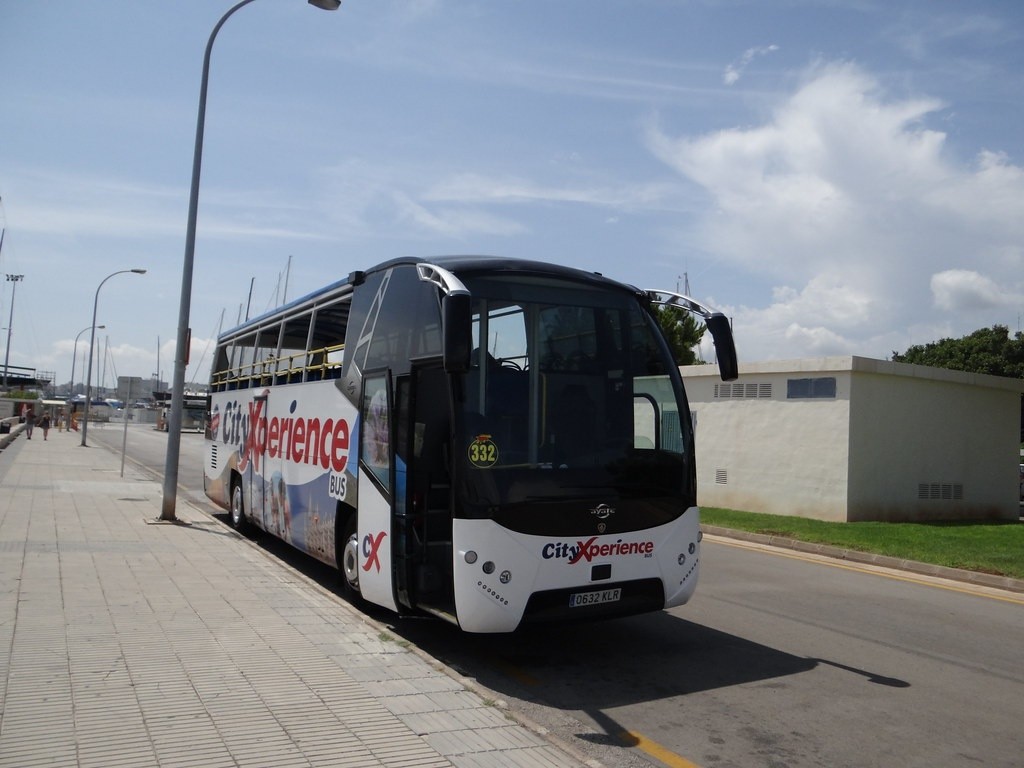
[
  {"x1": 58, "y1": 413, "x2": 64, "y2": 432},
  {"x1": 37, "y1": 411, "x2": 51, "y2": 440},
  {"x1": 25, "y1": 409, "x2": 37, "y2": 439}
]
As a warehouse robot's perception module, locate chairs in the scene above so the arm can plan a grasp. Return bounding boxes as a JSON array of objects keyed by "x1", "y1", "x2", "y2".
[
  {"x1": 541, "y1": 353, "x2": 599, "y2": 375},
  {"x1": 212, "y1": 347, "x2": 529, "y2": 482}
]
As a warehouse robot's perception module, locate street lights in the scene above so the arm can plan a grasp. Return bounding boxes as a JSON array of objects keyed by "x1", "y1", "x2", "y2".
[
  {"x1": 65, "y1": 324, "x2": 107, "y2": 432},
  {"x1": 158, "y1": 0, "x2": 343, "y2": 528},
  {"x1": 79, "y1": 267, "x2": 147, "y2": 448}
]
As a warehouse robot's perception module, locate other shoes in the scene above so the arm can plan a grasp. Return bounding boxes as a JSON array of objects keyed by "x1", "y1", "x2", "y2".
[
  {"x1": 27, "y1": 437, "x2": 32, "y2": 440},
  {"x1": 44, "y1": 438, "x2": 47, "y2": 441}
]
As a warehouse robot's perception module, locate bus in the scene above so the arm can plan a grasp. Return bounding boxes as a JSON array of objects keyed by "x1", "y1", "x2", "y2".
[
  {"x1": 71, "y1": 397, "x2": 114, "y2": 423},
  {"x1": 202, "y1": 250, "x2": 741, "y2": 644}
]
